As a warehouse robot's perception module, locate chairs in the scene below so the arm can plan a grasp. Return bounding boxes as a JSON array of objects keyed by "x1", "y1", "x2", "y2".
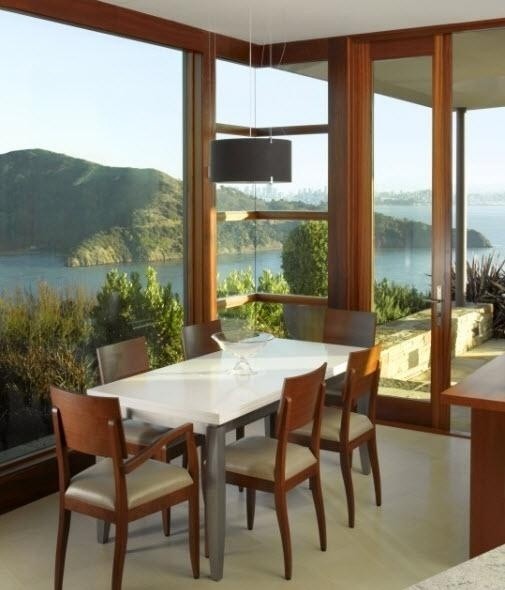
[{"x1": 45, "y1": 305, "x2": 383, "y2": 589}]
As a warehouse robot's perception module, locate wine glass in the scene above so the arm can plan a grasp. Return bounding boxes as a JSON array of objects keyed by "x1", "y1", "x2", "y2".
[{"x1": 211, "y1": 329, "x2": 274, "y2": 379}]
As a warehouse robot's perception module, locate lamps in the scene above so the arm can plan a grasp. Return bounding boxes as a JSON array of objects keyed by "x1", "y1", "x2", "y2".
[{"x1": 205, "y1": 1, "x2": 294, "y2": 186}]
